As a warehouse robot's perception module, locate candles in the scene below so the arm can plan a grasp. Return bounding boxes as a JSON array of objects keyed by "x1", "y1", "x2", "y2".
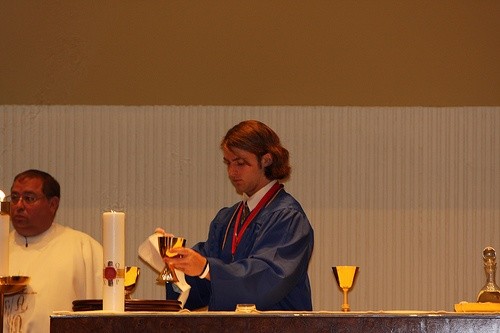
[{"x1": 102, "y1": 209, "x2": 125, "y2": 313}]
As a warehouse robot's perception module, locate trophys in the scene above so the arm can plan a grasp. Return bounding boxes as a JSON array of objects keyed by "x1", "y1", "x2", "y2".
[
  {"x1": 156, "y1": 236, "x2": 186, "y2": 282},
  {"x1": 124, "y1": 266, "x2": 141, "y2": 302},
  {"x1": 332, "y1": 266, "x2": 359, "y2": 312}
]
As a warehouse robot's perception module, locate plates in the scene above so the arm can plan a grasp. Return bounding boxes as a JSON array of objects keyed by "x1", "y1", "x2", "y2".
[{"x1": 0, "y1": 276, "x2": 29, "y2": 294}]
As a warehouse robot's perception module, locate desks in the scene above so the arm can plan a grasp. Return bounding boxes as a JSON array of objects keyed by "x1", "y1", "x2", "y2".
[{"x1": 49, "y1": 312, "x2": 500, "y2": 333}]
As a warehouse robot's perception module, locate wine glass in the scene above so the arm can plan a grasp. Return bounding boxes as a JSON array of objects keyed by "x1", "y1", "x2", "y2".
[
  {"x1": 123, "y1": 267, "x2": 141, "y2": 299},
  {"x1": 333, "y1": 266, "x2": 360, "y2": 312},
  {"x1": 156, "y1": 236, "x2": 186, "y2": 283}
]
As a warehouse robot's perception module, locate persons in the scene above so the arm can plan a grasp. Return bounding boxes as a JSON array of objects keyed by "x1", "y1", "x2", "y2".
[
  {"x1": 155, "y1": 120, "x2": 314, "y2": 313},
  {"x1": 0, "y1": 169, "x2": 103, "y2": 333}
]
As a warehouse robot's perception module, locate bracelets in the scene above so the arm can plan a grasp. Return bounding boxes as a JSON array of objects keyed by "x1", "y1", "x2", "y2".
[{"x1": 199, "y1": 257, "x2": 210, "y2": 279}]
